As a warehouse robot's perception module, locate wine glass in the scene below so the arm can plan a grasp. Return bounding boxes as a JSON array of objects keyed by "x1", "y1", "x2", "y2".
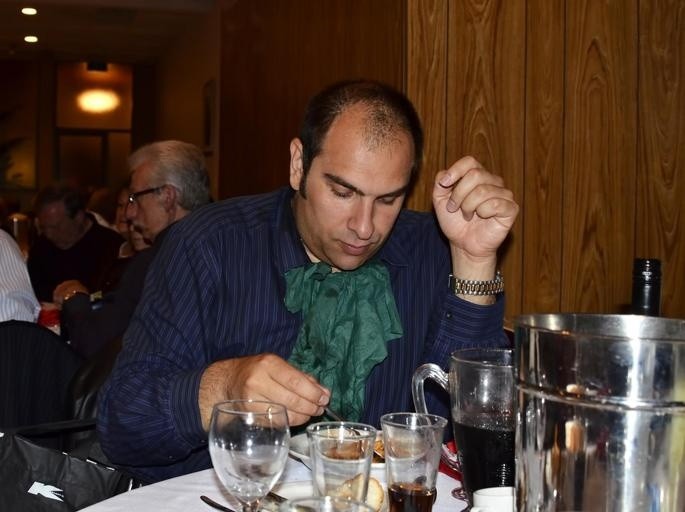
[{"x1": 207, "y1": 397, "x2": 290, "y2": 512}]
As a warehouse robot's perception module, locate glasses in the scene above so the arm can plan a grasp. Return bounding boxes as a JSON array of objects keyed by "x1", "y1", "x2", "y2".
[{"x1": 127, "y1": 187, "x2": 164, "y2": 201}]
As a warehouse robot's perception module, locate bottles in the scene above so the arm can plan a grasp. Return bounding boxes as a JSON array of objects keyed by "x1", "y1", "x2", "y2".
[{"x1": 631, "y1": 256, "x2": 661, "y2": 317}]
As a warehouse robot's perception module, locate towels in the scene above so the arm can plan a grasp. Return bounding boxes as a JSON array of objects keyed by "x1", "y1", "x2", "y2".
[{"x1": 282, "y1": 255, "x2": 404, "y2": 422}]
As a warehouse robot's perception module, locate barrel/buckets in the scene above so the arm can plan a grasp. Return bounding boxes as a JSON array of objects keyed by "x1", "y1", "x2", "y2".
[{"x1": 514, "y1": 313, "x2": 683, "y2": 512}]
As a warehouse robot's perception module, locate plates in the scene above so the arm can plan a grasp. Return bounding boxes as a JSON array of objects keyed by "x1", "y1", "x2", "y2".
[{"x1": 281, "y1": 422, "x2": 386, "y2": 473}]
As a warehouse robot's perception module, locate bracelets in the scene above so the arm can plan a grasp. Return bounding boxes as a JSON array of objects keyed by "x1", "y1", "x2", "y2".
[{"x1": 448, "y1": 271, "x2": 504, "y2": 295}]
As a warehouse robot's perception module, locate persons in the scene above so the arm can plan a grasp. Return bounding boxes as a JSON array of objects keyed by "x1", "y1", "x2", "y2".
[
  {"x1": 29, "y1": 141, "x2": 210, "y2": 367},
  {"x1": 2, "y1": 229, "x2": 39, "y2": 324},
  {"x1": 97, "y1": 80, "x2": 520, "y2": 487}
]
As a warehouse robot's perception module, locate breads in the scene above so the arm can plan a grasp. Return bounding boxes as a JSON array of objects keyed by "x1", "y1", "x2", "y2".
[{"x1": 327, "y1": 473, "x2": 384, "y2": 512}]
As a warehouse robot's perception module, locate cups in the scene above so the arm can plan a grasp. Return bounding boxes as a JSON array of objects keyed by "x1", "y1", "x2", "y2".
[
  {"x1": 306, "y1": 412, "x2": 447, "y2": 512},
  {"x1": 409, "y1": 345, "x2": 521, "y2": 509}
]
